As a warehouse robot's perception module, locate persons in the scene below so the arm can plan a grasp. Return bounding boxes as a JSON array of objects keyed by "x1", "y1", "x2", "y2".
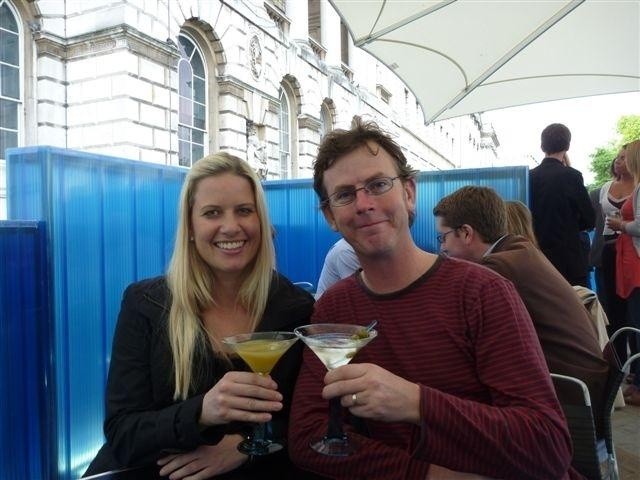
[
  {"x1": 81, "y1": 150, "x2": 316, "y2": 480},
  {"x1": 313, "y1": 237, "x2": 362, "y2": 301},
  {"x1": 529, "y1": 123, "x2": 640, "y2": 405},
  {"x1": 286, "y1": 122, "x2": 583, "y2": 479},
  {"x1": 503, "y1": 201, "x2": 538, "y2": 247},
  {"x1": 432, "y1": 186, "x2": 612, "y2": 439}
]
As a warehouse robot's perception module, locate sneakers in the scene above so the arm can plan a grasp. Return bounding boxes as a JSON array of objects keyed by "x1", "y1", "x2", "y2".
[{"x1": 623, "y1": 371, "x2": 640, "y2": 406}]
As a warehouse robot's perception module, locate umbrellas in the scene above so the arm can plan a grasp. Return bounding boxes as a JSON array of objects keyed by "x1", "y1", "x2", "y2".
[{"x1": 327, "y1": 0, "x2": 640, "y2": 127}]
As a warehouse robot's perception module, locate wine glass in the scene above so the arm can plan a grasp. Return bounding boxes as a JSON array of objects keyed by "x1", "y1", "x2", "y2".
[
  {"x1": 606, "y1": 208, "x2": 622, "y2": 238},
  {"x1": 294, "y1": 322, "x2": 379, "y2": 458},
  {"x1": 221, "y1": 330, "x2": 301, "y2": 458}
]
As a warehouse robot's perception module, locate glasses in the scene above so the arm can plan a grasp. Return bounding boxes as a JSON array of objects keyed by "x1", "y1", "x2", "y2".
[
  {"x1": 320, "y1": 174, "x2": 402, "y2": 207},
  {"x1": 436, "y1": 228, "x2": 457, "y2": 243}
]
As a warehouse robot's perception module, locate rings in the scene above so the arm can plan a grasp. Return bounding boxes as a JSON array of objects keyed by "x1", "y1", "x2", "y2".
[{"x1": 353, "y1": 393, "x2": 358, "y2": 406}]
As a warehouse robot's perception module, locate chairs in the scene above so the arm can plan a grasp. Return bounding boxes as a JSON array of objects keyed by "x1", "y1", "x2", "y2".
[
  {"x1": 550, "y1": 374, "x2": 602, "y2": 480},
  {"x1": 596, "y1": 326, "x2": 640, "y2": 480}
]
